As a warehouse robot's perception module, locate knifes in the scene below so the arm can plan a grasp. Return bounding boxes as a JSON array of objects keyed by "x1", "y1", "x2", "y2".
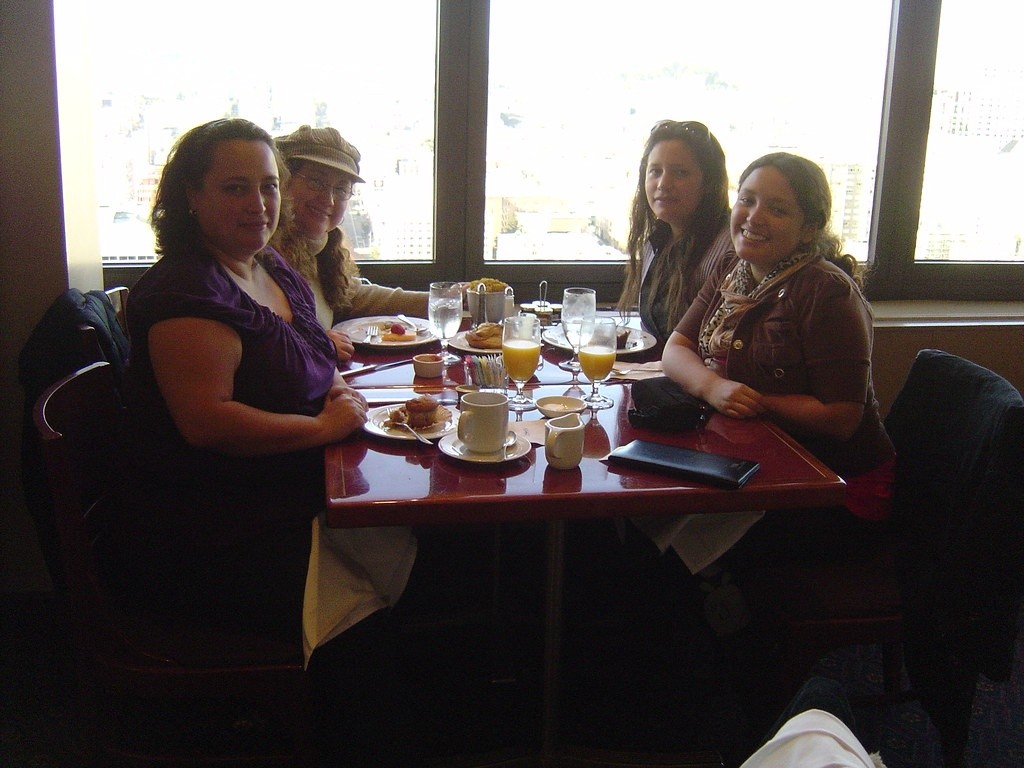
[{"x1": 338, "y1": 357, "x2": 414, "y2": 379}]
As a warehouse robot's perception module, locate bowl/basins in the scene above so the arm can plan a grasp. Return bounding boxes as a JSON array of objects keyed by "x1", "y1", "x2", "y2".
[{"x1": 467, "y1": 287, "x2": 512, "y2": 323}]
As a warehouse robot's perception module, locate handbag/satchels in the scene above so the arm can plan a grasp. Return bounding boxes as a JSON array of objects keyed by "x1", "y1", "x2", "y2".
[{"x1": 628, "y1": 378, "x2": 711, "y2": 431}]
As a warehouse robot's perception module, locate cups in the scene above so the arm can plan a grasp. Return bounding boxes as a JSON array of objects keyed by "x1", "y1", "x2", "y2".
[
  {"x1": 458, "y1": 392, "x2": 510, "y2": 453},
  {"x1": 413, "y1": 353, "x2": 443, "y2": 378}
]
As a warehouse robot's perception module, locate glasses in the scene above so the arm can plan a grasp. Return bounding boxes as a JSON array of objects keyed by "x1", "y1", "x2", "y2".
[
  {"x1": 651, "y1": 121, "x2": 712, "y2": 148},
  {"x1": 292, "y1": 169, "x2": 355, "y2": 200}
]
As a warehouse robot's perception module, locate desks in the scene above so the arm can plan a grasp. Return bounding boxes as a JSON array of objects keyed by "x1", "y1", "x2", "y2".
[{"x1": 325, "y1": 315, "x2": 845, "y2": 768}]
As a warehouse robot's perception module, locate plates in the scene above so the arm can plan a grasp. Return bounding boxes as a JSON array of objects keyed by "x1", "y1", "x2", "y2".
[
  {"x1": 437, "y1": 429, "x2": 532, "y2": 462},
  {"x1": 358, "y1": 401, "x2": 463, "y2": 439},
  {"x1": 536, "y1": 396, "x2": 588, "y2": 418},
  {"x1": 332, "y1": 313, "x2": 441, "y2": 347},
  {"x1": 447, "y1": 330, "x2": 546, "y2": 353},
  {"x1": 542, "y1": 321, "x2": 657, "y2": 356}
]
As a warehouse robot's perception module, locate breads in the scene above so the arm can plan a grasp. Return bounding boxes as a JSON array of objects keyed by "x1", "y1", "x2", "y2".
[
  {"x1": 405, "y1": 395, "x2": 439, "y2": 428},
  {"x1": 464, "y1": 322, "x2": 503, "y2": 348}
]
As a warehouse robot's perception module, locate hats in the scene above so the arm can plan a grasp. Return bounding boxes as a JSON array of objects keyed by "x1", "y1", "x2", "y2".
[{"x1": 272, "y1": 125, "x2": 366, "y2": 185}]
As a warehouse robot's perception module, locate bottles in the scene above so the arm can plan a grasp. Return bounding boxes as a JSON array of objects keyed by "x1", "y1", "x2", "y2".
[
  {"x1": 471, "y1": 283, "x2": 489, "y2": 327},
  {"x1": 498, "y1": 288, "x2": 515, "y2": 326},
  {"x1": 521, "y1": 299, "x2": 562, "y2": 327}
]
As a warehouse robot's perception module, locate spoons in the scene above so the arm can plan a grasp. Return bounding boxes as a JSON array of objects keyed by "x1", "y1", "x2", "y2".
[
  {"x1": 500, "y1": 431, "x2": 516, "y2": 461},
  {"x1": 397, "y1": 314, "x2": 428, "y2": 333}
]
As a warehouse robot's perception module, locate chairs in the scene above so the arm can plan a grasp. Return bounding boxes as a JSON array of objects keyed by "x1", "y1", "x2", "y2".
[
  {"x1": 781, "y1": 348, "x2": 1024, "y2": 768},
  {"x1": 18, "y1": 285, "x2": 318, "y2": 768}
]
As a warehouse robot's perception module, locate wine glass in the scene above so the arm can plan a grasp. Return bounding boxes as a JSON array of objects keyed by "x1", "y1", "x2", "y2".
[
  {"x1": 428, "y1": 281, "x2": 462, "y2": 365},
  {"x1": 582, "y1": 408, "x2": 610, "y2": 458},
  {"x1": 502, "y1": 316, "x2": 540, "y2": 412},
  {"x1": 562, "y1": 371, "x2": 587, "y2": 398},
  {"x1": 558, "y1": 286, "x2": 597, "y2": 372},
  {"x1": 577, "y1": 314, "x2": 617, "y2": 410}
]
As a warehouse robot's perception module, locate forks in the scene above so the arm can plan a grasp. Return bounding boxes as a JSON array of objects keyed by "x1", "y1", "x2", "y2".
[
  {"x1": 361, "y1": 324, "x2": 379, "y2": 345},
  {"x1": 386, "y1": 407, "x2": 435, "y2": 446},
  {"x1": 608, "y1": 365, "x2": 664, "y2": 377}
]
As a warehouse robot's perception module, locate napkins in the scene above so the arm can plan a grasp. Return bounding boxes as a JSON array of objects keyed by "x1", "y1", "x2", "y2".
[
  {"x1": 610, "y1": 362, "x2": 666, "y2": 381},
  {"x1": 505, "y1": 420, "x2": 550, "y2": 445}
]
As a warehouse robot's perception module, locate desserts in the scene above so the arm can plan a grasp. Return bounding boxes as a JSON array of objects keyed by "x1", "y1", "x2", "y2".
[{"x1": 380, "y1": 324, "x2": 416, "y2": 340}]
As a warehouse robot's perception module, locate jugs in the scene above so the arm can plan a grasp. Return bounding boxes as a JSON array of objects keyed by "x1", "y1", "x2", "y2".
[{"x1": 544, "y1": 412, "x2": 586, "y2": 470}]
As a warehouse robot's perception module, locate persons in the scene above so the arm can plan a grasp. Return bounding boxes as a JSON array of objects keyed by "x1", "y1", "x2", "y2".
[
  {"x1": 622, "y1": 152, "x2": 897, "y2": 768},
  {"x1": 118, "y1": 118, "x2": 399, "y2": 768},
  {"x1": 628, "y1": 119, "x2": 736, "y2": 370},
  {"x1": 263, "y1": 126, "x2": 470, "y2": 333}
]
